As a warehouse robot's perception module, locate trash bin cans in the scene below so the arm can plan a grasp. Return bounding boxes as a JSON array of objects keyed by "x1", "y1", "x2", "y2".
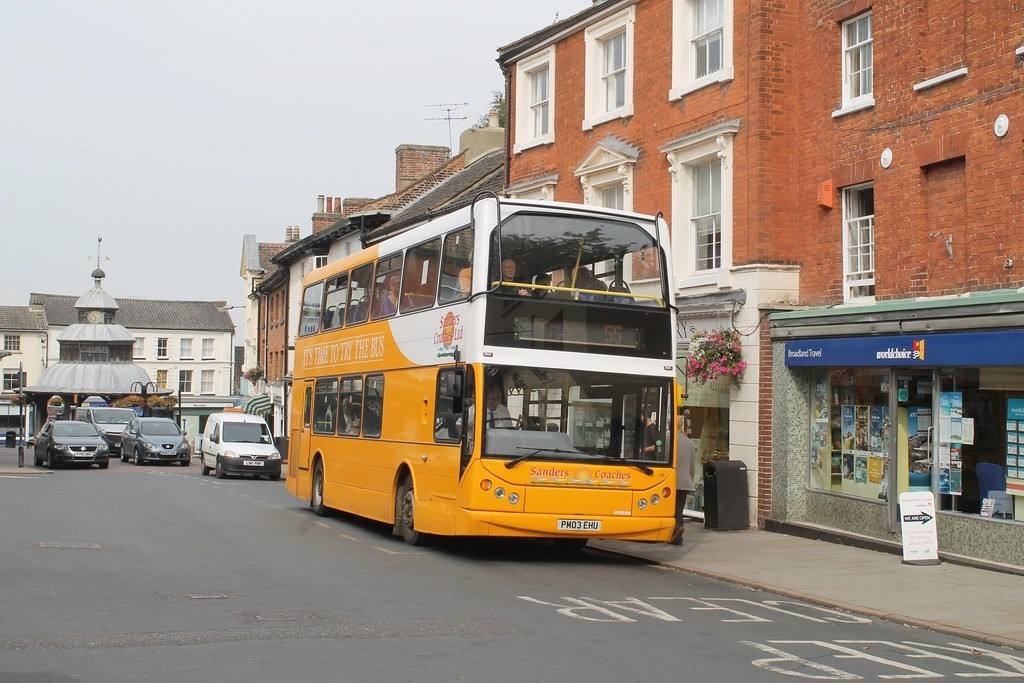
[
  {"x1": 702, "y1": 460, "x2": 750, "y2": 531},
  {"x1": 5, "y1": 430, "x2": 17, "y2": 448}
]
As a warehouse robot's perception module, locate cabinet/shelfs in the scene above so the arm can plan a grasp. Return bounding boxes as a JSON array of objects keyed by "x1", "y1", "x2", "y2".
[{"x1": 809, "y1": 373, "x2": 858, "y2": 491}]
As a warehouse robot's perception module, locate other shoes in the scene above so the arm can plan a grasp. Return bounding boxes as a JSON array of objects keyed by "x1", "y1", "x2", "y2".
[{"x1": 668, "y1": 523, "x2": 684, "y2": 544}]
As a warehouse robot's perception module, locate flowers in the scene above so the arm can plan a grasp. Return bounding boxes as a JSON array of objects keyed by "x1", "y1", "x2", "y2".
[
  {"x1": 244, "y1": 367, "x2": 264, "y2": 386},
  {"x1": 110, "y1": 394, "x2": 178, "y2": 408},
  {"x1": 9, "y1": 395, "x2": 27, "y2": 407},
  {"x1": 686, "y1": 327, "x2": 747, "y2": 390}
]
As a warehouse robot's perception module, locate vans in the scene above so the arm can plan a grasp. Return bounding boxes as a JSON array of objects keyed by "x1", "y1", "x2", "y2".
[
  {"x1": 75, "y1": 407, "x2": 138, "y2": 454},
  {"x1": 201, "y1": 412, "x2": 282, "y2": 481}
]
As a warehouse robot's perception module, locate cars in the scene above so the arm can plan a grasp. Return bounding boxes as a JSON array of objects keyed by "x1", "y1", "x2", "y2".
[{"x1": 120, "y1": 417, "x2": 191, "y2": 467}]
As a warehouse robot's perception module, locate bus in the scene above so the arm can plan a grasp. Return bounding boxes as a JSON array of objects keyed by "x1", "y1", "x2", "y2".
[{"x1": 283, "y1": 192, "x2": 691, "y2": 545}]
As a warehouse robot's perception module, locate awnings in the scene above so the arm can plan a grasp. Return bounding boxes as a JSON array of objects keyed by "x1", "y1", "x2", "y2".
[
  {"x1": 244, "y1": 395, "x2": 271, "y2": 415},
  {"x1": 675, "y1": 349, "x2": 729, "y2": 408}
]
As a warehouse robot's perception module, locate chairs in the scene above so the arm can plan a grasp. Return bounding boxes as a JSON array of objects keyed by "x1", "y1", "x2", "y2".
[
  {"x1": 332, "y1": 302, "x2": 346, "y2": 327},
  {"x1": 354, "y1": 295, "x2": 369, "y2": 321},
  {"x1": 326, "y1": 305, "x2": 337, "y2": 327},
  {"x1": 349, "y1": 299, "x2": 361, "y2": 323},
  {"x1": 975, "y1": 462, "x2": 1006, "y2": 509},
  {"x1": 555, "y1": 280, "x2": 637, "y2": 305},
  {"x1": 373, "y1": 291, "x2": 384, "y2": 318},
  {"x1": 380, "y1": 290, "x2": 392, "y2": 317}
]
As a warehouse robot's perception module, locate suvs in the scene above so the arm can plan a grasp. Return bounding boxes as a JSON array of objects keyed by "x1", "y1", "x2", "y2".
[{"x1": 34, "y1": 418, "x2": 110, "y2": 470}]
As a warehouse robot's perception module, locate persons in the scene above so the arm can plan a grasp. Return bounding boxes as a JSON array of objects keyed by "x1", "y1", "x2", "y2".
[
  {"x1": 334, "y1": 399, "x2": 360, "y2": 433},
  {"x1": 547, "y1": 423, "x2": 560, "y2": 433},
  {"x1": 871, "y1": 430, "x2": 879, "y2": 447},
  {"x1": 844, "y1": 432, "x2": 854, "y2": 450},
  {"x1": 665, "y1": 416, "x2": 695, "y2": 546},
  {"x1": 568, "y1": 266, "x2": 608, "y2": 302},
  {"x1": 856, "y1": 426, "x2": 864, "y2": 450},
  {"x1": 532, "y1": 275, "x2": 552, "y2": 299},
  {"x1": 349, "y1": 418, "x2": 361, "y2": 435},
  {"x1": 382, "y1": 276, "x2": 418, "y2": 315},
  {"x1": 639, "y1": 405, "x2": 661, "y2": 461},
  {"x1": 454, "y1": 251, "x2": 473, "y2": 297},
  {"x1": 820, "y1": 433, "x2": 826, "y2": 446},
  {"x1": 493, "y1": 258, "x2": 532, "y2": 297},
  {"x1": 456, "y1": 379, "x2": 513, "y2": 455}
]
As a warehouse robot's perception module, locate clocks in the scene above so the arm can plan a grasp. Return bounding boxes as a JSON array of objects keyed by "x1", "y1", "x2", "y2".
[{"x1": 87, "y1": 310, "x2": 103, "y2": 324}]
